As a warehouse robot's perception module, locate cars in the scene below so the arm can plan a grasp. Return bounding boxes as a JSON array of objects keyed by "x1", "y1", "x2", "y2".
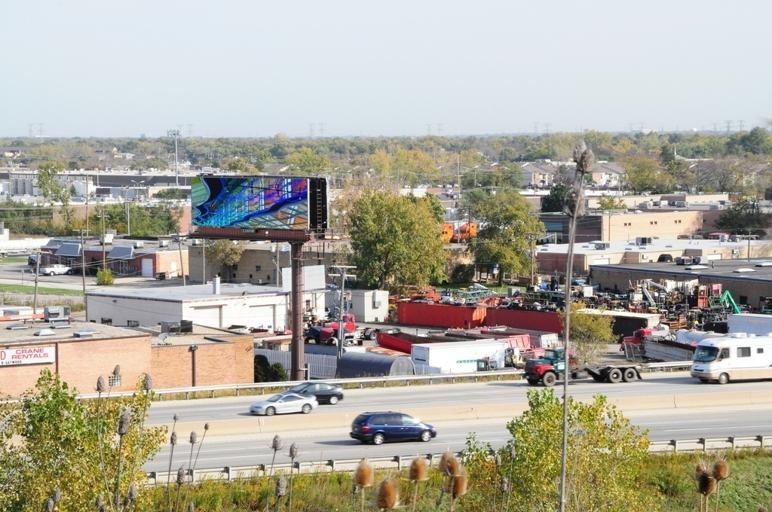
[
  {"x1": 250, "y1": 382, "x2": 343, "y2": 416},
  {"x1": 39, "y1": 263, "x2": 76, "y2": 275}
]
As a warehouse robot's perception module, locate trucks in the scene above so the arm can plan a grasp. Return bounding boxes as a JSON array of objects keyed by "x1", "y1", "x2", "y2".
[
  {"x1": 523, "y1": 348, "x2": 641, "y2": 386},
  {"x1": 319, "y1": 313, "x2": 402, "y2": 345}
]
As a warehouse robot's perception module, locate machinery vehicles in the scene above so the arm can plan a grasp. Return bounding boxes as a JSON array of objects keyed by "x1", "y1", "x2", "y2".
[{"x1": 626, "y1": 278, "x2": 741, "y2": 332}]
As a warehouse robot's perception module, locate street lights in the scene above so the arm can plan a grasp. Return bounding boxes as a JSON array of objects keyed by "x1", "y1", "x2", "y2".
[
  {"x1": 130, "y1": 178, "x2": 144, "y2": 200},
  {"x1": 167, "y1": 127, "x2": 183, "y2": 186}
]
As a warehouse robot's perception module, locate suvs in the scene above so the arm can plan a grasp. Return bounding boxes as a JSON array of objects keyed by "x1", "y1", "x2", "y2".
[{"x1": 350, "y1": 410, "x2": 436, "y2": 444}]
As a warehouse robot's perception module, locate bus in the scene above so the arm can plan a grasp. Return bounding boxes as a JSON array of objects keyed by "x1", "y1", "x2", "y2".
[{"x1": 691, "y1": 337, "x2": 772, "y2": 384}]
